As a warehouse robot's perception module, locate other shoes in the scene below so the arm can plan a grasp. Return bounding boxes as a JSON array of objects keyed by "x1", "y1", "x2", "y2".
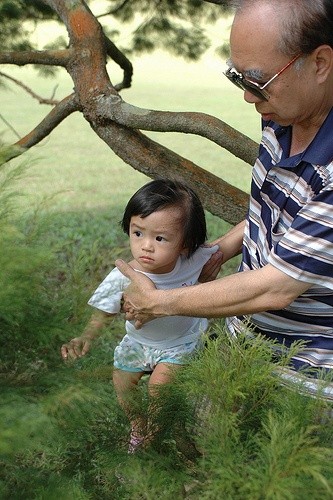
[{"x1": 127, "y1": 432, "x2": 146, "y2": 456}]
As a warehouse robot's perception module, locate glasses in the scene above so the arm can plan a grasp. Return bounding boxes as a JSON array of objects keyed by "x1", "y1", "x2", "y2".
[{"x1": 222, "y1": 52, "x2": 304, "y2": 102}]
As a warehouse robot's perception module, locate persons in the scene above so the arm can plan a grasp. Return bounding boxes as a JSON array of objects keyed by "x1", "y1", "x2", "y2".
[
  {"x1": 59, "y1": 179, "x2": 247, "y2": 462},
  {"x1": 114, "y1": 1, "x2": 332, "y2": 448}
]
